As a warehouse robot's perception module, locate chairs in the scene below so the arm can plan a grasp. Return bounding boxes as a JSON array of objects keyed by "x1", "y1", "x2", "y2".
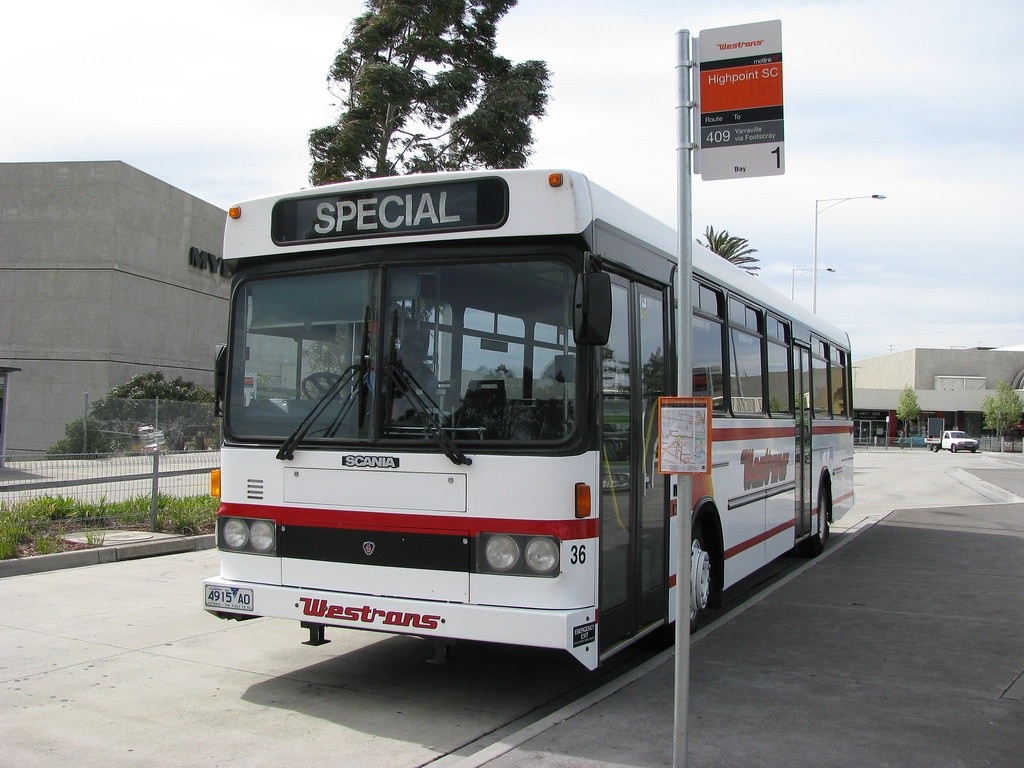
[{"x1": 457, "y1": 380, "x2": 572, "y2": 440}]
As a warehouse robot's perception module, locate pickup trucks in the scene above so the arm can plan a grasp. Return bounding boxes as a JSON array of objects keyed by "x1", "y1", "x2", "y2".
[{"x1": 924, "y1": 429, "x2": 980, "y2": 453}]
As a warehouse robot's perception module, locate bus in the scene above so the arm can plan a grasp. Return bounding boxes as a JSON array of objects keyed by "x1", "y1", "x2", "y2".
[
  {"x1": 200, "y1": 167, "x2": 856, "y2": 674},
  {"x1": 604, "y1": 388, "x2": 664, "y2": 493}
]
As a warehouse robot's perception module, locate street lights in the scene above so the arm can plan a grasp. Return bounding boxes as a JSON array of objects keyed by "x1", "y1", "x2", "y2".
[
  {"x1": 790, "y1": 268, "x2": 835, "y2": 302},
  {"x1": 812, "y1": 194, "x2": 889, "y2": 316}
]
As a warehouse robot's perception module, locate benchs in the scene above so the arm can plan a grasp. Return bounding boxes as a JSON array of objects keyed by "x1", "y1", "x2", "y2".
[{"x1": 896, "y1": 437, "x2": 925, "y2": 447}]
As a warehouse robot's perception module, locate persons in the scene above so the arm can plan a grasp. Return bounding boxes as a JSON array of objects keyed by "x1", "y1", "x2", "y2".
[
  {"x1": 898, "y1": 430, "x2": 905, "y2": 449},
  {"x1": 364, "y1": 329, "x2": 438, "y2": 422}
]
